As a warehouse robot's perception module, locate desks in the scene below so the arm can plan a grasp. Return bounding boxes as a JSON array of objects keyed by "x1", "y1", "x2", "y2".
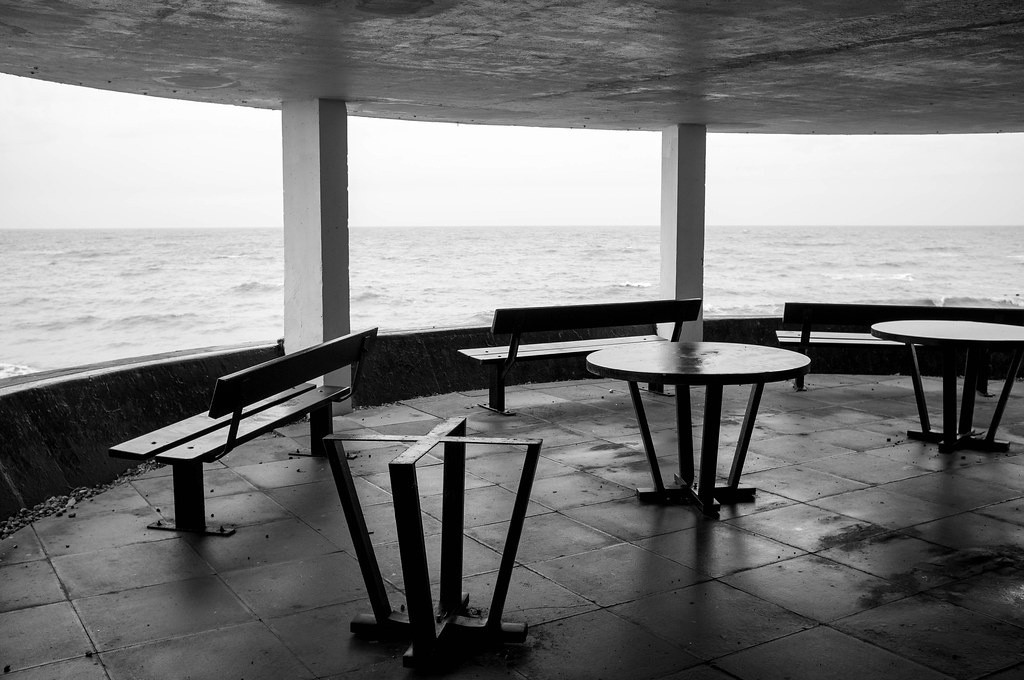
[
  {"x1": 870, "y1": 320, "x2": 1024, "y2": 453},
  {"x1": 586, "y1": 342, "x2": 811, "y2": 514}
]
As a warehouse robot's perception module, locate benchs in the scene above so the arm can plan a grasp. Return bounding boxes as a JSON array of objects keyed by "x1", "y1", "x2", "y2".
[
  {"x1": 457, "y1": 297, "x2": 704, "y2": 417},
  {"x1": 108, "y1": 325, "x2": 381, "y2": 537},
  {"x1": 775, "y1": 303, "x2": 1024, "y2": 399}
]
